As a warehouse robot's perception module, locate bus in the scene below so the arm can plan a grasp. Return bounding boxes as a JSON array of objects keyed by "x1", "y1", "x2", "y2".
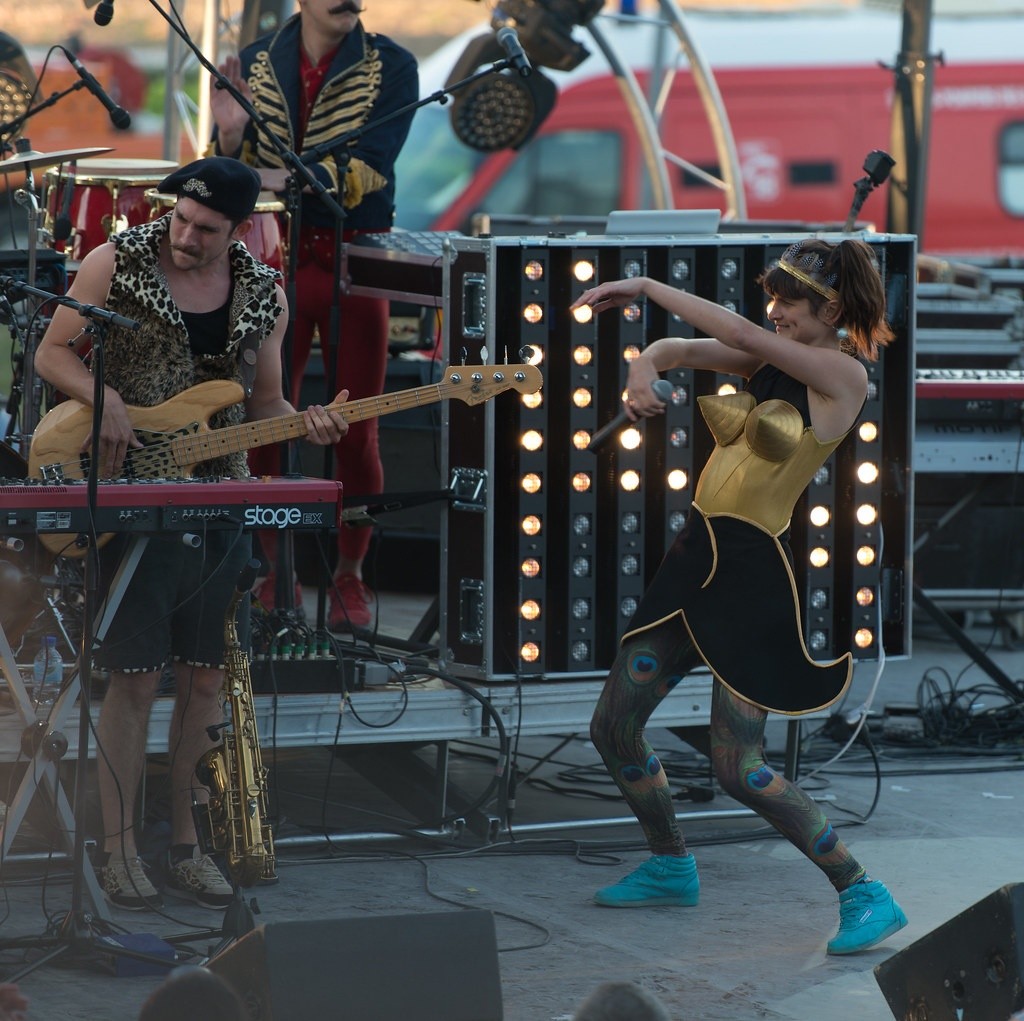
[{"x1": 396, "y1": 12, "x2": 1023, "y2": 270}]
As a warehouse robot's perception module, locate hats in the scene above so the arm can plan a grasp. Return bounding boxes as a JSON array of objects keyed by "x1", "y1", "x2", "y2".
[{"x1": 156, "y1": 156, "x2": 262, "y2": 220}]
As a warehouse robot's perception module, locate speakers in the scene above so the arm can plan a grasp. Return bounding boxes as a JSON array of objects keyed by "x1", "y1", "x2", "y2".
[
  {"x1": 872, "y1": 882, "x2": 1024, "y2": 1021},
  {"x1": 207, "y1": 911, "x2": 508, "y2": 1021}
]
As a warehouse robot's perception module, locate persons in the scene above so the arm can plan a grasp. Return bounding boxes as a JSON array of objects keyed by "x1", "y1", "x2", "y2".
[
  {"x1": 202, "y1": 1, "x2": 421, "y2": 637},
  {"x1": 573, "y1": 979, "x2": 671, "y2": 1021},
  {"x1": 138, "y1": 964, "x2": 252, "y2": 1021},
  {"x1": 567, "y1": 239, "x2": 910, "y2": 955},
  {"x1": 26, "y1": 158, "x2": 350, "y2": 912}
]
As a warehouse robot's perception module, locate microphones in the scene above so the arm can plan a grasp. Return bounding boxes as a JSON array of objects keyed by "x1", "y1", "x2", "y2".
[
  {"x1": 587, "y1": 379, "x2": 673, "y2": 453},
  {"x1": 0, "y1": 293, "x2": 13, "y2": 316},
  {"x1": 94, "y1": 0, "x2": 114, "y2": 27},
  {"x1": 54, "y1": 161, "x2": 77, "y2": 241},
  {"x1": 65, "y1": 50, "x2": 130, "y2": 128},
  {"x1": 497, "y1": 26, "x2": 533, "y2": 77}
]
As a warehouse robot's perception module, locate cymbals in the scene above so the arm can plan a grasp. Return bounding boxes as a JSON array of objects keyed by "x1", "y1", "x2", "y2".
[{"x1": 0, "y1": 146, "x2": 115, "y2": 173}]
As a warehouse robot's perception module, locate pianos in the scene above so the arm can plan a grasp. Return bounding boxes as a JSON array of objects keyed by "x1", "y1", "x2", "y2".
[{"x1": 0, "y1": 474, "x2": 342, "y2": 916}]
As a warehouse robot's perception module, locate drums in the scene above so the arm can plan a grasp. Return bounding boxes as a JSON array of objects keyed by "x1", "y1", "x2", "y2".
[
  {"x1": 32, "y1": 157, "x2": 181, "y2": 319},
  {"x1": 143, "y1": 185, "x2": 286, "y2": 296}
]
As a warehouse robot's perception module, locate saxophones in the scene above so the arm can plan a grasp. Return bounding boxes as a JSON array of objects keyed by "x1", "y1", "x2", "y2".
[{"x1": 195, "y1": 559, "x2": 279, "y2": 885}]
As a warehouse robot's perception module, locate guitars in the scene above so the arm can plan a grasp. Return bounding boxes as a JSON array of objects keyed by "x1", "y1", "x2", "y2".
[{"x1": 28, "y1": 344, "x2": 544, "y2": 559}]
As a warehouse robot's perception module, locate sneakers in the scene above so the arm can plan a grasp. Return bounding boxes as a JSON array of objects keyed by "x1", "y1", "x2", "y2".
[
  {"x1": 325, "y1": 575, "x2": 375, "y2": 633},
  {"x1": 592, "y1": 852, "x2": 701, "y2": 908},
  {"x1": 827, "y1": 879, "x2": 908, "y2": 955},
  {"x1": 163, "y1": 846, "x2": 234, "y2": 910},
  {"x1": 99, "y1": 856, "x2": 165, "y2": 912},
  {"x1": 260, "y1": 575, "x2": 302, "y2": 614}
]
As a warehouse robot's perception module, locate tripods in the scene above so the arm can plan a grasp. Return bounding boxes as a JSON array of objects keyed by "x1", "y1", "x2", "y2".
[{"x1": 0, "y1": 275, "x2": 254, "y2": 990}]
